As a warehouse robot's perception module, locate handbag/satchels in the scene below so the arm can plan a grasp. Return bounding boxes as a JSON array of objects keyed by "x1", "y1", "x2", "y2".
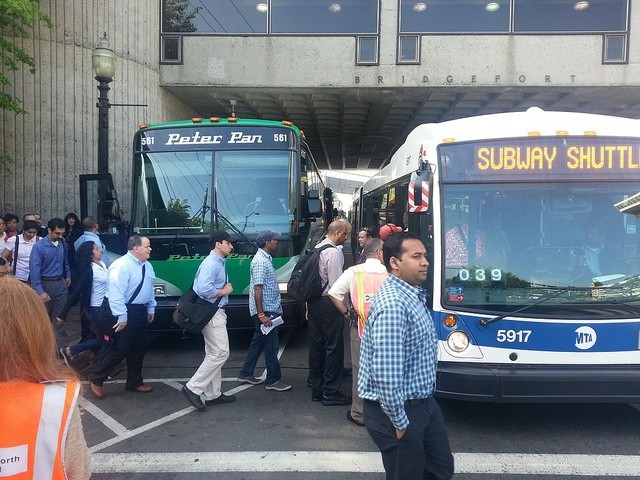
[
  {"x1": 173, "y1": 289, "x2": 219, "y2": 333},
  {"x1": 96, "y1": 296, "x2": 119, "y2": 344}
]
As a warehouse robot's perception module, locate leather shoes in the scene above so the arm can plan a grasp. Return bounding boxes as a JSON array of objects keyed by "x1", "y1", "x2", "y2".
[
  {"x1": 312, "y1": 397, "x2": 323, "y2": 401},
  {"x1": 307, "y1": 378, "x2": 313, "y2": 387},
  {"x1": 322, "y1": 393, "x2": 352, "y2": 405},
  {"x1": 90, "y1": 382, "x2": 103, "y2": 397},
  {"x1": 343, "y1": 366, "x2": 352, "y2": 376},
  {"x1": 205, "y1": 394, "x2": 236, "y2": 406},
  {"x1": 134, "y1": 386, "x2": 152, "y2": 392},
  {"x1": 182, "y1": 385, "x2": 205, "y2": 410}
]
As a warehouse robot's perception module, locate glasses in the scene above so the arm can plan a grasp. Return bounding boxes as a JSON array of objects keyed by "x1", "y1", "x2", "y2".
[
  {"x1": 54, "y1": 232, "x2": 66, "y2": 236},
  {"x1": 360, "y1": 227, "x2": 369, "y2": 231}
]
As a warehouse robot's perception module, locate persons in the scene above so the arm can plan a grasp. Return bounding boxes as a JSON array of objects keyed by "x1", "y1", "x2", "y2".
[
  {"x1": 74, "y1": 216, "x2": 111, "y2": 270},
  {"x1": 575, "y1": 224, "x2": 617, "y2": 279},
  {"x1": 4, "y1": 214, "x2": 19, "y2": 242},
  {"x1": 34, "y1": 214, "x2": 41, "y2": 221},
  {"x1": 29, "y1": 217, "x2": 71, "y2": 328},
  {"x1": 1, "y1": 276, "x2": 91, "y2": 480},
  {"x1": 336, "y1": 219, "x2": 352, "y2": 272},
  {"x1": 0, "y1": 218, "x2": 7, "y2": 257},
  {"x1": 23, "y1": 214, "x2": 37, "y2": 223},
  {"x1": 309, "y1": 220, "x2": 353, "y2": 406},
  {"x1": 357, "y1": 229, "x2": 372, "y2": 264},
  {"x1": 64, "y1": 213, "x2": 84, "y2": 246},
  {"x1": 55, "y1": 241, "x2": 108, "y2": 363},
  {"x1": 357, "y1": 233, "x2": 454, "y2": 480},
  {"x1": 1, "y1": 220, "x2": 44, "y2": 282},
  {"x1": 182, "y1": 231, "x2": 236, "y2": 409},
  {"x1": 328, "y1": 238, "x2": 388, "y2": 426},
  {"x1": 238, "y1": 230, "x2": 292, "y2": 391},
  {"x1": 88, "y1": 235, "x2": 157, "y2": 397}
]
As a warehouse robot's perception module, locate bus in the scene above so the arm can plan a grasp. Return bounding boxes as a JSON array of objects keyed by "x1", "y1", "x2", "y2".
[
  {"x1": 349, "y1": 107, "x2": 640, "y2": 406},
  {"x1": 78, "y1": 115, "x2": 335, "y2": 342}
]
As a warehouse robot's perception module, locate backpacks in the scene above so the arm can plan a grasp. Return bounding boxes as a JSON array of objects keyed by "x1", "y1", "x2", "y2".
[{"x1": 287, "y1": 244, "x2": 335, "y2": 302}]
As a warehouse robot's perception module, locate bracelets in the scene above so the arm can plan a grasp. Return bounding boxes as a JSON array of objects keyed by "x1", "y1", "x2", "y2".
[
  {"x1": 344, "y1": 309, "x2": 350, "y2": 316},
  {"x1": 258, "y1": 313, "x2": 264, "y2": 318}
]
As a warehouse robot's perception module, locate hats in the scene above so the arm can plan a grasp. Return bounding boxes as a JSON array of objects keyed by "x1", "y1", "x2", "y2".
[
  {"x1": 256, "y1": 230, "x2": 281, "y2": 246},
  {"x1": 210, "y1": 230, "x2": 239, "y2": 242}
]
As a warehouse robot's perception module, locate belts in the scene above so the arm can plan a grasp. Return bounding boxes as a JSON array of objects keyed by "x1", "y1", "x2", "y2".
[{"x1": 41, "y1": 278, "x2": 61, "y2": 281}]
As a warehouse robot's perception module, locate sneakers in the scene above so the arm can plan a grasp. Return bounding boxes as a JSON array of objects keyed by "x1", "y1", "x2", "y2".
[
  {"x1": 238, "y1": 376, "x2": 263, "y2": 385},
  {"x1": 347, "y1": 411, "x2": 365, "y2": 426},
  {"x1": 265, "y1": 381, "x2": 292, "y2": 391},
  {"x1": 60, "y1": 347, "x2": 73, "y2": 368}
]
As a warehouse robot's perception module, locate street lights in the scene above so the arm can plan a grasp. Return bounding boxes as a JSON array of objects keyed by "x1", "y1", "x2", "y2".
[{"x1": 90, "y1": 31, "x2": 119, "y2": 237}]
